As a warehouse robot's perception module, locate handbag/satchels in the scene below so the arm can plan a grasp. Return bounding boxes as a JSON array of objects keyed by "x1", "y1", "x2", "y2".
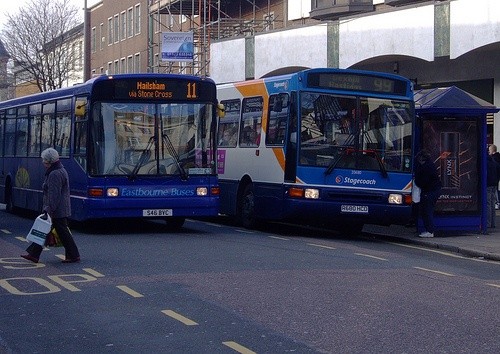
[
  {"x1": 420, "y1": 185, "x2": 441, "y2": 204},
  {"x1": 48, "y1": 225, "x2": 73, "y2": 248},
  {"x1": 27, "y1": 212, "x2": 52, "y2": 246},
  {"x1": 412, "y1": 182, "x2": 422, "y2": 203}
]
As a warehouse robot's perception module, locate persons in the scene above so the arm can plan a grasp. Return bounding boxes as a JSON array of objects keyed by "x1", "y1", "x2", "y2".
[
  {"x1": 220, "y1": 125, "x2": 260, "y2": 147},
  {"x1": 415, "y1": 152, "x2": 442, "y2": 237},
  {"x1": 484, "y1": 148, "x2": 498, "y2": 227},
  {"x1": 21, "y1": 148, "x2": 81, "y2": 264},
  {"x1": 489, "y1": 145, "x2": 500, "y2": 209}
]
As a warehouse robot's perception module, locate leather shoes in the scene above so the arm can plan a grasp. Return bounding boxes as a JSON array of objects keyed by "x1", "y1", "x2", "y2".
[
  {"x1": 61, "y1": 257, "x2": 79, "y2": 264},
  {"x1": 20, "y1": 253, "x2": 39, "y2": 264}
]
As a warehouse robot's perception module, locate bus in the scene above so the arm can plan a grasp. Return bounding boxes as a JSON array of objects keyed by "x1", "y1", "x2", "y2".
[
  {"x1": 215, "y1": 67, "x2": 416, "y2": 233},
  {"x1": 0, "y1": 73, "x2": 225, "y2": 228}
]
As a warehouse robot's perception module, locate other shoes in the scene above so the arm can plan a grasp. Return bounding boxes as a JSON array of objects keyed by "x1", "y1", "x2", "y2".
[{"x1": 419, "y1": 231, "x2": 434, "y2": 238}]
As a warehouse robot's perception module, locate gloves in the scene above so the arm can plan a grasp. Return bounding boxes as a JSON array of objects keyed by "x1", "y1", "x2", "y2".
[{"x1": 42, "y1": 211, "x2": 47, "y2": 215}]
